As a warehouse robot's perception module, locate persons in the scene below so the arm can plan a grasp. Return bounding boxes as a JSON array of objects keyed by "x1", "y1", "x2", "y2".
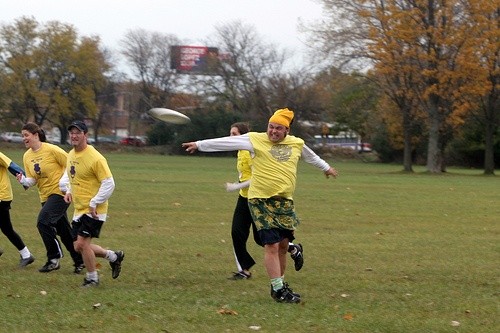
[
  {"x1": 64, "y1": 120, "x2": 124, "y2": 287},
  {"x1": 181, "y1": 108, "x2": 338, "y2": 303},
  {"x1": 0, "y1": 122, "x2": 86, "y2": 273}
]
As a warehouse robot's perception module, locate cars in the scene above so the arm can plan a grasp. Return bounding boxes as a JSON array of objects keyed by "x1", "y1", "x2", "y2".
[{"x1": 0, "y1": 126, "x2": 147, "y2": 147}]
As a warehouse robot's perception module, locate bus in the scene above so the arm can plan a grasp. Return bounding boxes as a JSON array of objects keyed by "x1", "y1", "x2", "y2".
[{"x1": 298, "y1": 120, "x2": 372, "y2": 154}]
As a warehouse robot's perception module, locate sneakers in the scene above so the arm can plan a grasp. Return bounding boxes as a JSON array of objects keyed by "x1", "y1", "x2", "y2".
[
  {"x1": 290, "y1": 242, "x2": 304, "y2": 271},
  {"x1": 109, "y1": 250, "x2": 124, "y2": 278},
  {"x1": 73, "y1": 263, "x2": 86, "y2": 273},
  {"x1": 38, "y1": 260, "x2": 61, "y2": 273},
  {"x1": 19, "y1": 253, "x2": 34, "y2": 267},
  {"x1": 228, "y1": 271, "x2": 252, "y2": 280},
  {"x1": 83, "y1": 274, "x2": 99, "y2": 287},
  {"x1": 270, "y1": 282, "x2": 300, "y2": 303}
]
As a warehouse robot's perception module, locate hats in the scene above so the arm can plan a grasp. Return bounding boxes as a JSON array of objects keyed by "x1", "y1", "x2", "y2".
[
  {"x1": 68, "y1": 120, "x2": 88, "y2": 135},
  {"x1": 269, "y1": 108, "x2": 294, "y2": 128}
]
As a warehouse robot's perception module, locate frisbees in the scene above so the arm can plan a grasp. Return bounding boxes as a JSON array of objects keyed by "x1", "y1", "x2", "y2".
[{"x1": 149, "y1": 108, "x2": 191, "y2": 124}]
